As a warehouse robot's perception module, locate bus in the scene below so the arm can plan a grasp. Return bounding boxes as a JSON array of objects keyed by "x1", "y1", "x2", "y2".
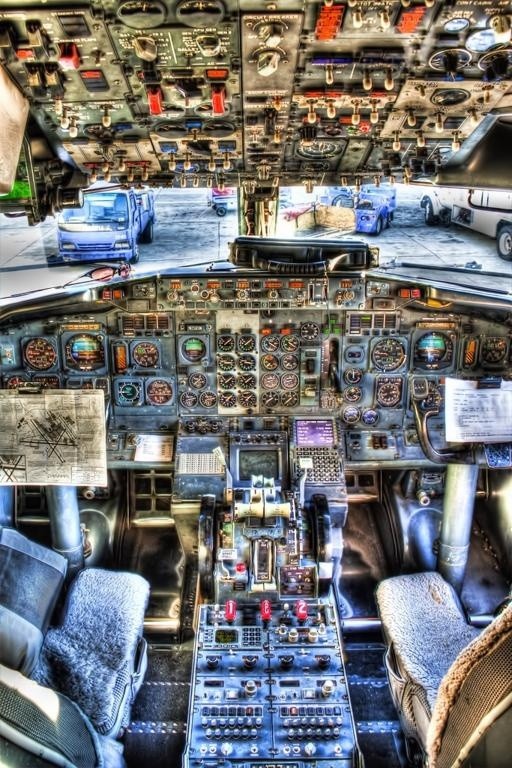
[{"x1": 420, "y1": 187, "x2": 512, "y2": 261}]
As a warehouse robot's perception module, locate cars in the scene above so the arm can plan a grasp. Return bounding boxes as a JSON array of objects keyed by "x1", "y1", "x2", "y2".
[
  {"x1": 322, "y1": 183, "x2": 396, "y2": 236},
  {"x1": 207, "y1": 187, "x2": 294, "y2": 217}
]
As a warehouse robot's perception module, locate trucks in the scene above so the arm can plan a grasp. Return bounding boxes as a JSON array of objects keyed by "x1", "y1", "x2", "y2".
[{"x1": 57, "y1": 186, "x2": 156, "y2": 264}]
